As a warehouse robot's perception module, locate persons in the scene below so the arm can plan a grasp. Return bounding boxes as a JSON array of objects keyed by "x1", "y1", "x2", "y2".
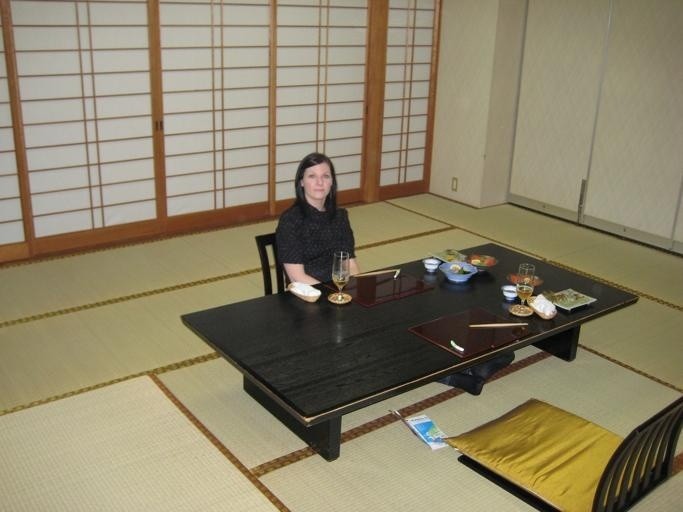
[{"x1": 275, "y1": 152, "x2": 359, "y2": 286}]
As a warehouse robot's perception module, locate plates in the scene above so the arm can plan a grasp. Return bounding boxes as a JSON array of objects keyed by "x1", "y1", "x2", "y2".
[
  {"x1": 429, "y1": 249, "x2": 467, "y2": 263},
  {"x1": 545, "y1": 288, "x2": 597, "y2": 312}
]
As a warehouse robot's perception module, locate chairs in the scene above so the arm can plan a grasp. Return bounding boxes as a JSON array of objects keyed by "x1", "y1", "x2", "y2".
[
  {"x1": 447, "y1": 394, "x2": 683, "y2": 512},
  {"x1": 257, "y1": 233, "x2": 291, "y2": 298}
]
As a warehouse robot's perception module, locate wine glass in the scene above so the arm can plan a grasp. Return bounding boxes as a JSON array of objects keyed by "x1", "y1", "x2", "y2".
[
  {"x1": 516, "y1": 263, "x2": 536, "y2": 314},
  {"x1": 332, "y1": 251, "x2": 350, "y2": 302}
]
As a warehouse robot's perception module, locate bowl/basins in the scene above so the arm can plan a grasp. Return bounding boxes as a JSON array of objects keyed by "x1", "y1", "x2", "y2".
[
  {"x1": 502, "y1": 285, "x2": 520, "y2": 301},
  {"x1": 422, "y1": 254, "x2": 499, "y2": 282}
]
